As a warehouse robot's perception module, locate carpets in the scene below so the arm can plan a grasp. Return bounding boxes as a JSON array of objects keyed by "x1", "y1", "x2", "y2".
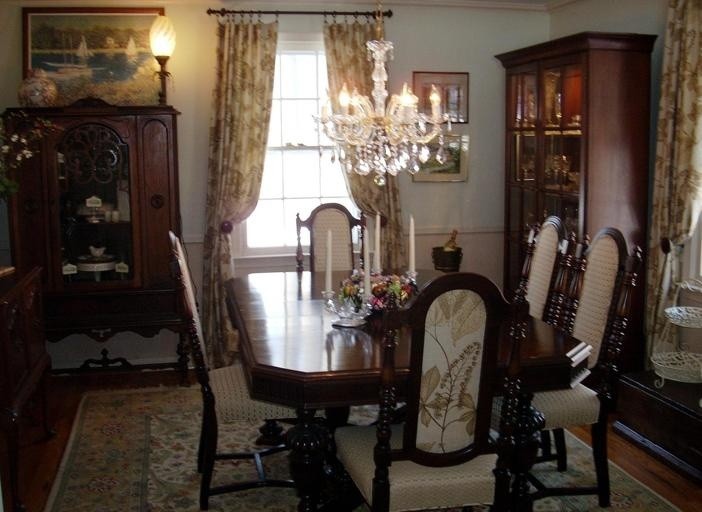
[{"x1": 42, "y1": 383, "x2": 679, "y2": 512}]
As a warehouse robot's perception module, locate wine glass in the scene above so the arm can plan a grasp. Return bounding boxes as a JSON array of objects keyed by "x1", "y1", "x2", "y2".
[
  {"x1": 545, "y1": 154, "x2": 570, "y2": 187},
  {"x1": 521, "y1": 154, "x2": 535, "y2": 179}
]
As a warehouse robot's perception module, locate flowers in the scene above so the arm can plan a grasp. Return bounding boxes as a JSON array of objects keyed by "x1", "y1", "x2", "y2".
[{"x1": 337, "y1": 267, "x2": 416, "y2": 312}]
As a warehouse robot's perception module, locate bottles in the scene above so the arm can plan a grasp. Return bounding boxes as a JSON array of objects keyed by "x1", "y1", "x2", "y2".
[{"x1": 443, "y1": 229, "x2": 458, "y2": 251}]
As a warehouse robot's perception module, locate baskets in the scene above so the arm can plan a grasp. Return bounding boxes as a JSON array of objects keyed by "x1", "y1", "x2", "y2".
[
  {"x1": 664, "y1": 307, "x2": 701, "y2": 329},
  {"x1": 650, "y1": 351, "x2": 701, "y2": 384}
]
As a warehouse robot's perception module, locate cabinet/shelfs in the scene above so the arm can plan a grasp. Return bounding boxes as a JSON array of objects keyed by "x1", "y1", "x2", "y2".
[
  {"x1": 0, "y1": 264, "x2": 55, "y2": 512},
  {"x1": 614, "y1": 369, "x2": 700, "y2": 484},
  {"x1": 4, "y1": 106, "x2": 190, "y2": 385},
  {"x1": 494, "y1": 32, "x2": 657, "y2": 303}
]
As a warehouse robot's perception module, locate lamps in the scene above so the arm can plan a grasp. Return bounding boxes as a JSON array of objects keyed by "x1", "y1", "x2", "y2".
[
  {"x1": 314, "y1": 1, "x2": 461, "y2": 186},
  {"x1": 148, "y1": 16, "x2": 175, "y2": 107}
]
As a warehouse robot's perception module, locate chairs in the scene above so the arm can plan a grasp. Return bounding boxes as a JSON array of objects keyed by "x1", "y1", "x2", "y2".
[
  {"x1": 520, "y1": 216, "x2": 568, "y2": 324},
  {"x1": 332, "y1": 270, "x2": 543, "y2": 512},
  {"x1": 295, "y1": 202, "x2": 369, "y2": 270},
  {"x1": 168, "y1": 231, "x2": 328, "y2": 510},
  {"x1": 491, "y1": 229, "x2": 628, "y2": 509}
]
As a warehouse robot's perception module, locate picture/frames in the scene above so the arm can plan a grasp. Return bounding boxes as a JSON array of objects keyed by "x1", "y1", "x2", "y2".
[
  {"x1": 411, "y1": 135, "x2": 471, "y2": 183},
  {"x1": 411, "y1": 70, "x2": 470, "y2": 124},
  {"x1": 22, "y1": 8, "x2": 164, "y2": 106}
]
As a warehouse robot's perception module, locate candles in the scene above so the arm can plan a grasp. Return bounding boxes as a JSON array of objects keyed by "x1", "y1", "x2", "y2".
[
  {"x1": 362, "y1": 225, "x2": 372, "y2": 297},
  {"x1": 373, "y1": 211, "x2": 381, "y2": 270},
  {"x1": 323, "y1": 232, "x2": 333, "y2": 293},
  {"x1": 409, "y1": 214, "x2": 416, "y2": 274}
]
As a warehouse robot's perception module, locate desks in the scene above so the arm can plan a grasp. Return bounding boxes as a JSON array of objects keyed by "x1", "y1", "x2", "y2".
[{"x1": 228, "y1": 269, "x2": 588, "y2": 512}]
